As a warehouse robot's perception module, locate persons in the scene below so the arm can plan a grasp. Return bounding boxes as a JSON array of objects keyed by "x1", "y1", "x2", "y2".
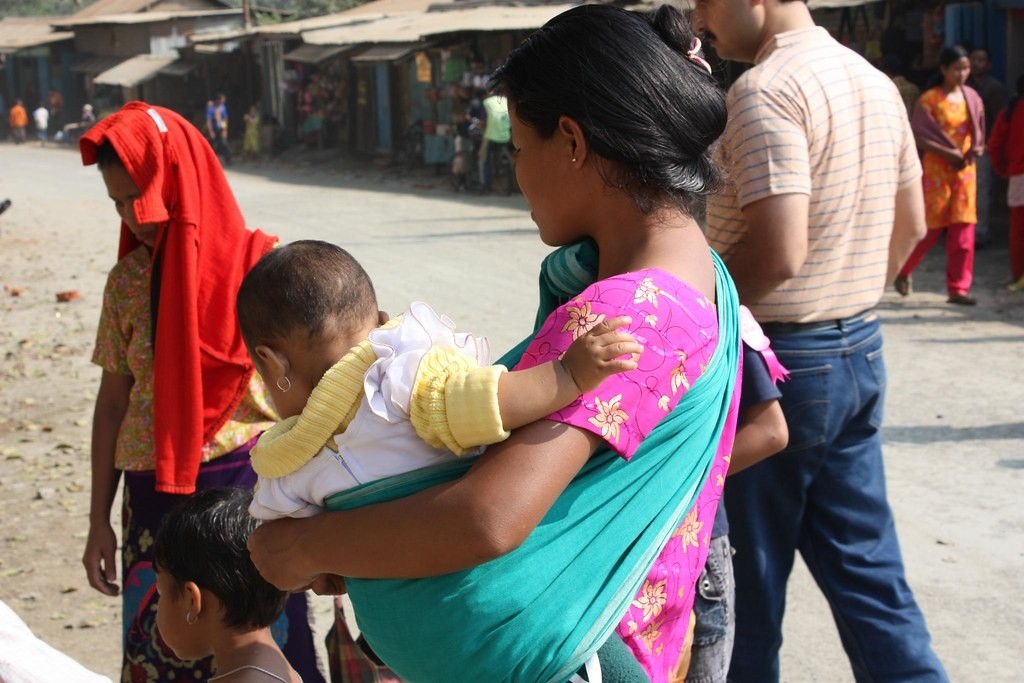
[
  {"x1": 248, "y1": 0, "x2": 744, "y2": 683},
  {"x1": 10, "y1": 81, "x2": 513, "y2": 195},
  {"x1": 152, "y1": 485, "x2": 303, "y2": 683},
  {"x1": 236, "y1": 239, "x2": 657, "y2": 683},
  {"x1": 688, "y1": 0, "x2": 950, "y2": 683},
  {"x1": 685, "y1": 305, "x2": 789, "y2": 683},
  {"x1": 882, "y1": 46, "x2": 1024, "y2": 306},
  {"x1": 79, "y1": 100, "x2": 325, "y2": 683}
]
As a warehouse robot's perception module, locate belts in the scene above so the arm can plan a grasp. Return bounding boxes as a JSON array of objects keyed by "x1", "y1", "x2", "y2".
[{"x1": 759, "y1": 308, "x2": 877, "y2": 336}]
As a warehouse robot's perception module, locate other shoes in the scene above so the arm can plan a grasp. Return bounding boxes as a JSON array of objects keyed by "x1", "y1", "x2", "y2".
[
  {"x1": 945, "y1": 293, "x2": 976, "y2": 306},
  {"x1": 894, "y1": 274, "x2": 913, "y2": 296}
]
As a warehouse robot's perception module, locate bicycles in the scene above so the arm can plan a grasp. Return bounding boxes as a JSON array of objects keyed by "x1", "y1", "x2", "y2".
[{"x1": 463, "y1": 130, "x2": 516, "y2": 198}]
